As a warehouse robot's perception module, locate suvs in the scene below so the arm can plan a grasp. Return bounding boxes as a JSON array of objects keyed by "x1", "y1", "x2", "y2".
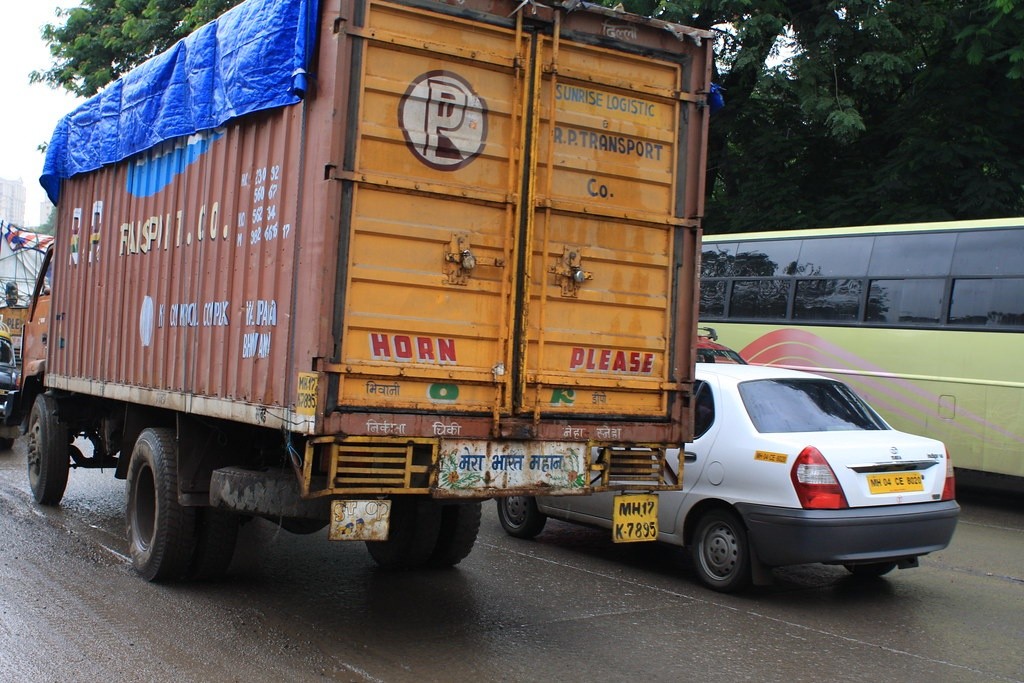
[{"x1": 696, "y1": 329, "x2": 746, "y2": 367}]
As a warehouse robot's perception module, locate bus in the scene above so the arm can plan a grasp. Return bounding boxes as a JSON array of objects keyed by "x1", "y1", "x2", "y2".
[{"x1": 694, "y1": 214, "x2": 1024, "y2": 507}]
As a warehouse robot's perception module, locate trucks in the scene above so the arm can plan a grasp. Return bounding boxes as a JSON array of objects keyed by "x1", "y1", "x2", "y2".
[{"x1": 4, "y1": 0, "x2": 718, "y2": 589}]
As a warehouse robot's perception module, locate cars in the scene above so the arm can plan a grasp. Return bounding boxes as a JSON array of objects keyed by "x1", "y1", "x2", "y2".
[
  {"x1": 493, "y1": 361, "x2": 961, "y2": 598},
  {"x1": 0, "y1": 319, "x2": 25, "y2": 451}
]
